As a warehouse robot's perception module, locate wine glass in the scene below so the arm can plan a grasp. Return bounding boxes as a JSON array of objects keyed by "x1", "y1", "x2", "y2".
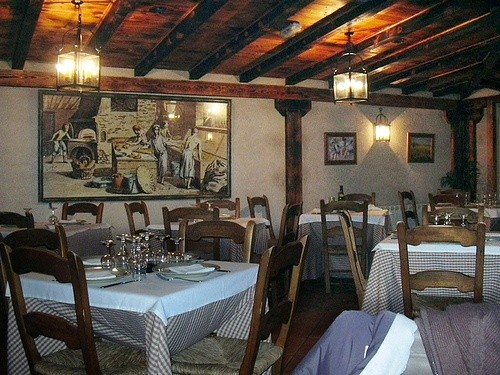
[
  {"x1": 48, "y1": 207, "x2": 57, "y2": 225},
  {"x1": 482, "y1": 192, "x2": 497, "y2": 206},
  {"x1": 473, "y1": 193, "x2": 481, "y2": 203},
  {"x1": 100, "y1": 232, "x2": 184, "y2": 280},
  {"x1": 431, "y1": 212, "x2": 468, "y2": 227}
]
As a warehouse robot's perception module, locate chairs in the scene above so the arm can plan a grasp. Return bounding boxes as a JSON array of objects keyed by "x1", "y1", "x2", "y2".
[{"x1": 0, "y1": 189, "x2": 488, "y2": 375}]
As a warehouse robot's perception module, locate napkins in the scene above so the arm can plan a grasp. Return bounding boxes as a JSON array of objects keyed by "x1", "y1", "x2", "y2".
[
  {"x1": 86, "y1": 271, "x2": 116, "y2": 281},
  {"x1": 168, "y1": 263, "x2": 215, "y2": 274}
]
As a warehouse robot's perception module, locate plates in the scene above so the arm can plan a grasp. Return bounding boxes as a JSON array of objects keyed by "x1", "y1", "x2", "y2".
[
  {"x1": 219, "y1": 214, "x2": 231, "y2": 219},
  {"x1": 83, "y1": 258, "x2": 101, "y2": 267},
  {"x1": 85, "y1": 270, "x2": 116, "y2": 280},
  {"x1": 153, "y1": 264, "x2": 220, "y2": 278}
]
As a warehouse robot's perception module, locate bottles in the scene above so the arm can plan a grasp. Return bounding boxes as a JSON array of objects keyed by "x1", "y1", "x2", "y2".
[
  {"x1": 463, "y1": 192, "x2": 469, "y2": 205},
  {"x1": 338, "y1": 185, "x2": 344, "y2": 213},
  {"x1": 329, "y1": 196, "x2": 335, "y2": 213}
]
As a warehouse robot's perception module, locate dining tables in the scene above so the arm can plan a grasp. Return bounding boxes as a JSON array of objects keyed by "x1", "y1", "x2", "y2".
[
  {"x1": 435, "y1": 203, "x2": 500, "y2": 231},
  {"x1": 4, "y1": 255, "x2": 272, "y2": 375},
  {"x1": 146, "y1": 217, "x2": 270, "y2": 264},
  {"x1": 297, "y1": 209, "x2": 393, "y2": 281},
  {"x1": 361, "y1": 233, "x2": 500, "y2": 318},
  {"x1": 0, "y1": 222, "x2": 118, "y2": 261}
]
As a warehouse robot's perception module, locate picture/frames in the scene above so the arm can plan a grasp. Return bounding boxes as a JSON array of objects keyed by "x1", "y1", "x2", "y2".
[
  {"x1": 406, "y1": 132, "x2": 435, "y2": 163},
  {"x1": 37, "y1": 90, "x2": 232, "y2": 199},
  {"x1": 324, "y1": 132, "x2": 357, "y2": 165}
]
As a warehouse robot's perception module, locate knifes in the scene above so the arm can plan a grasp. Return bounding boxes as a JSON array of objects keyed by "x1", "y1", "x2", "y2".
[{"x1": 100, "y1": 278, "x2": 141, "y2": 288}]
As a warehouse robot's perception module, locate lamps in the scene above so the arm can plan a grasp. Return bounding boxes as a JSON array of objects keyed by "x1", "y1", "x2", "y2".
[
  {"x1": 332, "y1": 28, "x2": 369, "y2": 105},
  {"x1": 373, "y1": 107, "x2": 391, "y2": 143},
  {"x1": 55, "y1": 0, "x2": 101, "y2": 93}
]
